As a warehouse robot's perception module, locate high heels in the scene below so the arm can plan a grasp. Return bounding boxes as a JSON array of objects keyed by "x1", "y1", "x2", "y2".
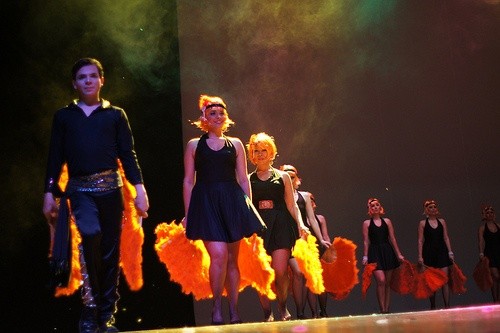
[{"x1": 211, "y1": 309, "x2": 225, "y2": 325}]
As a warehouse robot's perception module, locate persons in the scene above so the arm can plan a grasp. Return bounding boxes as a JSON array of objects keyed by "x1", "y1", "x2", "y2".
[
  {"x1": 478, "y1": 203, "x2": 500, "y2": 304},
  {"x1": 42, "y1": 58, "x2": 149, "y2": 333},
  {"x1": 416, "y1": 200, "x2": 455, "y2": 310},
  {"x1": 180, "y1": 94, "x2": 330, "y2": 325},
  {"x1": 361, "y1": 197, "x2": 405, "y2": 314}
]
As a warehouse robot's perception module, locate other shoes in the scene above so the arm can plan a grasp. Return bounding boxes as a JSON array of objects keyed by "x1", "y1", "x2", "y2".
[
  {"x1": 98, "y1": 323, "x2": 119, "y2": 333},
  {"x1": 79, "y1": 313, "x2": 95, "y2": 333}
]
